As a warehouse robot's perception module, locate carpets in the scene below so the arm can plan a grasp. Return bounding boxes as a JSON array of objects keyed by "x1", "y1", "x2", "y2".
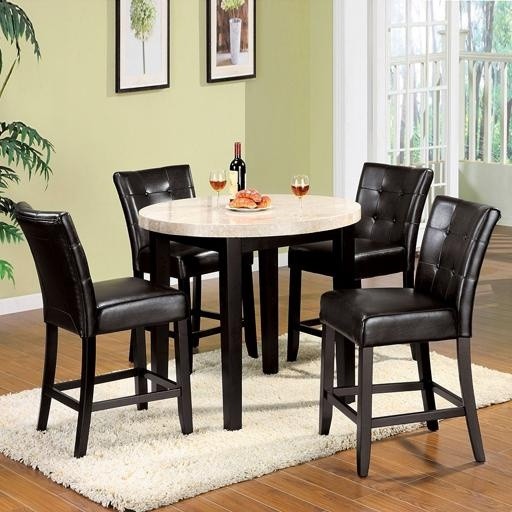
[{"x1": 1, "y1": 328, "x2": 512, "y2": 509}]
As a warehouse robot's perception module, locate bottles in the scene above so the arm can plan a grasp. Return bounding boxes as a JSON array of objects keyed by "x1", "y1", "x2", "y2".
[{"x1": 228, "y1": 141, "x2": 246, "y2": 203}]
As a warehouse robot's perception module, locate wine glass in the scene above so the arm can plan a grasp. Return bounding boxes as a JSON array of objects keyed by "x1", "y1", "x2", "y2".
[
  {"x1": 209, "y1": 169, "x2": 227, "y2": 210},
  {"x1": 291, "y1": 175, "x2": 310, "y2": 211}
]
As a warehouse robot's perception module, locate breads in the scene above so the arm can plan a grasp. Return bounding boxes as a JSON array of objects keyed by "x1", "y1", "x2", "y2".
[
  {"x1": 236, "y1": 188, "x2": 262, "y2": 203},
  {"x1": 229, "y1": 197, "x2": 257, "y2": 209},
  {"x1": 257, "y1": 195, "x2": 272, "y2": 208}
]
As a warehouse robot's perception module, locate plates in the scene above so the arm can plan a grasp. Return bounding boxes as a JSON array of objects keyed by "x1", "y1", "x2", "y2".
[{"x1": 225, "y1": 203, "x2": 273, "y2": 213}]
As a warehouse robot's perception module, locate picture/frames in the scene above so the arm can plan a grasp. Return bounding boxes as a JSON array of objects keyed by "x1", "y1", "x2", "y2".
[
  {"x1": 203, "y1": 0, "x2": 258, "y2": 85},
  {"x1": 113, "y1": 0, "x2": 173, "y2": 94}
]
